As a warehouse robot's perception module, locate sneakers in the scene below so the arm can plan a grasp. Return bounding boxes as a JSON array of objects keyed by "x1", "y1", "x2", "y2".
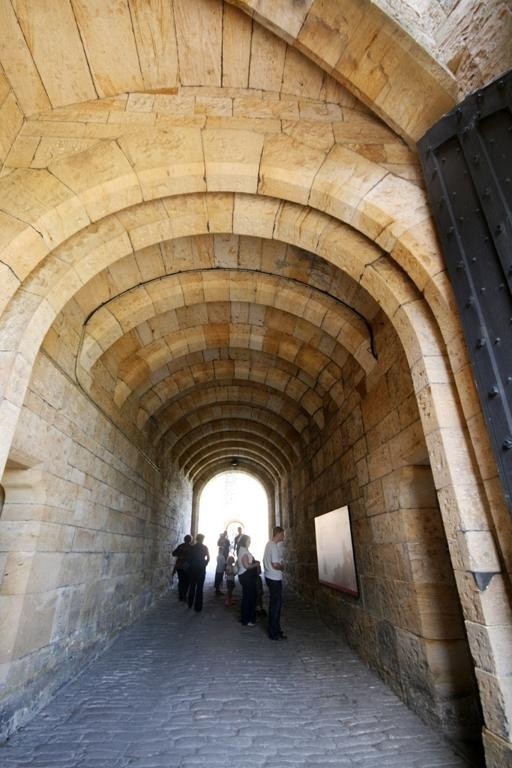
[
  {"x1": 270, "y1": 631, "x2": 287, "y2": 641},
  {"x1": 241, "y1": 619, "x2": 256, "y2": 627}
]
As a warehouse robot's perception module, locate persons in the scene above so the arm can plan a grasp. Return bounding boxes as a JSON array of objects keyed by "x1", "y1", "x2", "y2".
[
  {"x1": 171, "y1": 527, "x2": 261, "y2": 629},
  {"x1": 264, "y1": 527, "x2": 287, "y2": 642}
]
